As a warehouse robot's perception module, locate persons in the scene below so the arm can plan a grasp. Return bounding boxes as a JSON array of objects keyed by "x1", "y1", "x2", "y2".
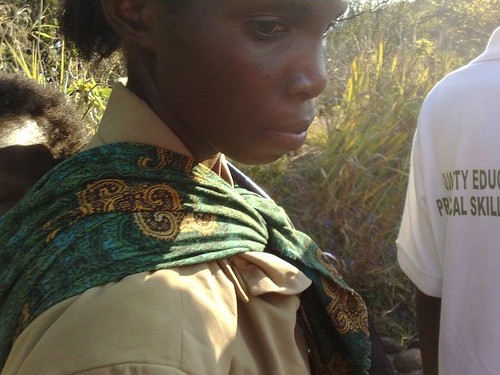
[
  {"x1": 0, "y1": 0, "x2": 372, "y2": 375},
  {"x1": 396, "y1": 25, "x2": 500, "y2": 375}
]
